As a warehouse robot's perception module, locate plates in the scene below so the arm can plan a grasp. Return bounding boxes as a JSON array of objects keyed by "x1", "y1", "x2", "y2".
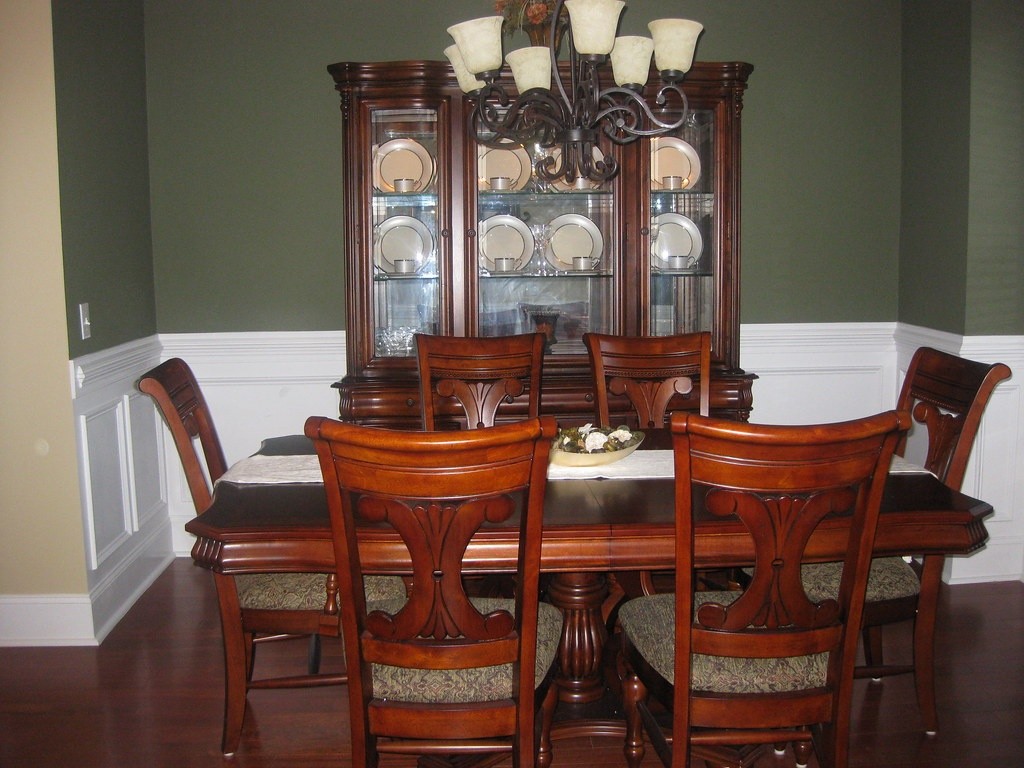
[
  {"x1": 372, "y1": 216, "x2": 434, "y2": 278},
  {"x1": 545, "y1": 140, "x2": 607, "y2": 192},
  {"x1": 476, "y1": 138, "x2": 532, "y2": 192},
  {"x1": 372, "y1": 137, "x2": 434, "y2": 195},
  {"x1": 478, "y1": 214, "x2": 535, "y2": 275},
  {"x1": 543, "y1": 212, "x2": 606, "y2": 275},
  {"x1": 650, "y1": 137, "x2": 701, "y2": 193},
  {"x1": 650, "y1": 213, "x2": 704, "y2": 274}
]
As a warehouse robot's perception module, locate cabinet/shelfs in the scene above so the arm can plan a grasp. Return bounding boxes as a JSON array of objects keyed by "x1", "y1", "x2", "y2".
[{"x1": 326, "y1": 60, "x2": 759, "y2": 430}]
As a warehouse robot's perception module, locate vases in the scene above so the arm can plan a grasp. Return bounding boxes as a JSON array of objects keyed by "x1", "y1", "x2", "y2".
[{"x1": 524, "y1": 22, "x2": 568, "y2": 61}]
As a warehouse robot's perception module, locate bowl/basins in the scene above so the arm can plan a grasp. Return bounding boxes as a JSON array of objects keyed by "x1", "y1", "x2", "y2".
[{"x1": 548, "y1": 430, "x2": 646, "y2": 467}]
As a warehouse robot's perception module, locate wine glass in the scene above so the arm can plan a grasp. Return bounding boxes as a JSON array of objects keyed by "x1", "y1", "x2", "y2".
[
  {"x1": 374, "y1": 324, "x2": 422, "y2": 357},
  {"x1": 528, "y1": 222, "x2": 552, "y2": 275},
  {"x1": 530, "y1": 311, "x2": 557, "y2": 355}
]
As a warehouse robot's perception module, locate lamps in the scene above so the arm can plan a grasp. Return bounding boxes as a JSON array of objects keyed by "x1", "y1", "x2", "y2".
[{"x1": 442, "y1": 0, "x2": 704, "y2": 184}]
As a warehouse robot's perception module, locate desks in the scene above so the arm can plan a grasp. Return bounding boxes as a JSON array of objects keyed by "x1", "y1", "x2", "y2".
[{"x1": 184, "y1": 429, "x2": 996, "y2": 768}]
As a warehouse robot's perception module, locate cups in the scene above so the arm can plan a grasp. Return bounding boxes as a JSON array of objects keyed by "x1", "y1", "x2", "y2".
[
  {"x1": 569, "y1": 174, "x2": 590, "y2": 188},
  {"x1": 661, "y1": 176, "x2": 690, "y2": 190},
  {"x1": 393, "y1": 179, "x2": 423, "y2": 192},
  {"x1": 489, "y1": 175, "x2": 517, "y2": 189},
  {"x1": 393, "y1": 258, "x2": 422, "y2": 272},
  {"x1": 667, "y1": 256, "x2": 696, "y2": 269},
  {"x1": 495, "y1": 255, "x2": 523, "y2": 271},
  {"x1": 570, "y1": 254, "x2": 600, "y2": 270}
]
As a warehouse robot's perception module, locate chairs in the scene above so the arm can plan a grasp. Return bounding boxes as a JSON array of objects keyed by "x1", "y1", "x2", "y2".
[{"x1": 136, "y1": 331, "x2": 1014, "y2": 768}]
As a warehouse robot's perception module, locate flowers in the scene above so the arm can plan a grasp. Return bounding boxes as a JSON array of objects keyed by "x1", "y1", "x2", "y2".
[{"x1": 494, "y1": 0, "x2": 571, "y2": 57}]
